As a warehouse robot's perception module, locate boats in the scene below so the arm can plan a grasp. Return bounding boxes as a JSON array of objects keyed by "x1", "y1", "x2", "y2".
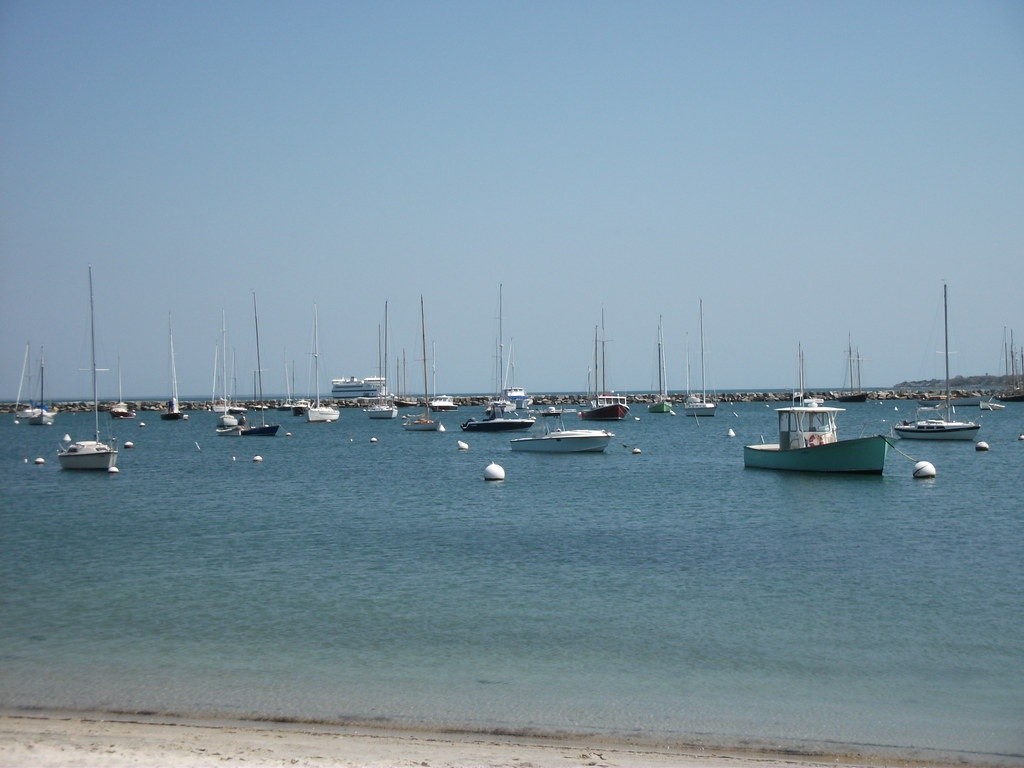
[
  {"x1": 332, "y1": 376, "x2": 388, "y2": 399},
  {"x1": 510, "y1": 409, "x2": 615, "y2": 453},
  {"x1": 743, "y1": 397, "x2": 898, "y2": 475},
  {"x1": 429, "y1": 394, "x2": 458, "y2": 412},
  {"x1": 917, "y1": 395, "x2": 995, "y2": 406},
  {"x1": 460, "y1": 403, "x2": 535, "y2": 432}
]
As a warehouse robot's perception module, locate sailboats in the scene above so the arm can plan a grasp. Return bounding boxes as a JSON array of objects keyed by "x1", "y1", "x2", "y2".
[
  {"x1": 793, "y1": 341, "x2": 806, "y2": 403},
  {"x1": 684, "y1": 298, "x2": 719, "y2": 416},
  {"x1": 893, "y1": 284, "x2": 982, "y2": 440},
  {"x1": 683, "y1": 332, "x2": 701, "y2": 403},
  {"x1": 652, "y1": 314, "x2": 672, "y2": 402},
  {"x1": 485, "y1": 283, "x2": 532, "y2": 415},
  {"x1": 648, "y1": 325, "x2": 672, "y2": 413},
  {"x1": 581, "y1": 326, "x2": 630, "y2": 420},
  {"x1": 590, "y1": 308, "x2": 627, "y2": 408},
  {"x1": 991, "y1": 326, "x2": 1024, "y2": 402},
  {"x1": 14, "y1": 288, "x2": 417, "y2": 437},
  {"x1": 402, "y1": 295, "x2": 442, "y2": 431},
  {"x1": 56, "y1": 264, "x2": 120, "y2": 470},
  {"x1": 836, "y1": 331, "x2": 868, "y2": 402}
]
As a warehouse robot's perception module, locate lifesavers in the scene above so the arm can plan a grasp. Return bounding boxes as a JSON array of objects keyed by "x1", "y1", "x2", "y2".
[{"x1": 809, "y1": 435, "x2": 823, "y2": 449}]
